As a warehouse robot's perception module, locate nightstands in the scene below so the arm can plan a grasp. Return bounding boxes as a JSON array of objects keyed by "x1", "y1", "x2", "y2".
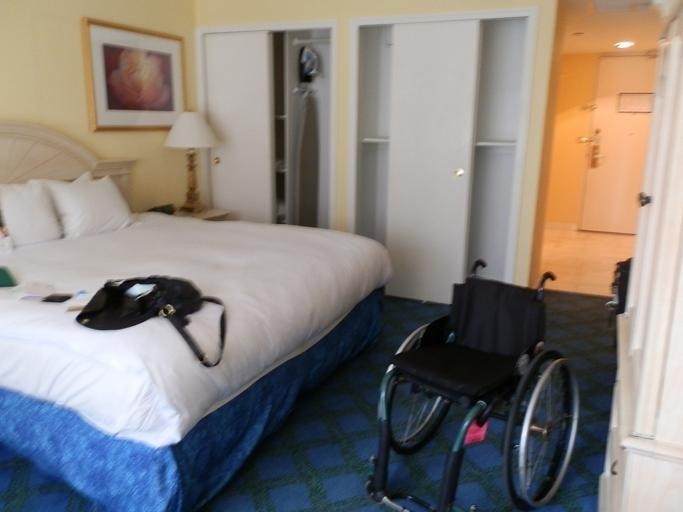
[{"x1": 171, "y1": 205, "x2": 231, "y2": 221}]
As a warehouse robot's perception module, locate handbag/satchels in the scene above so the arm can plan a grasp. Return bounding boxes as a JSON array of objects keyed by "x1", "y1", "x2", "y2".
[{"x1": 77, "y1": 277, "x2": 229, "y2": 369}]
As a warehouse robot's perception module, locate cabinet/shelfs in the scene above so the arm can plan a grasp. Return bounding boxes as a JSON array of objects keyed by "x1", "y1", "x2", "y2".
[{"x1": 270, "y1": 28, "x2": 329, "y2": 229}]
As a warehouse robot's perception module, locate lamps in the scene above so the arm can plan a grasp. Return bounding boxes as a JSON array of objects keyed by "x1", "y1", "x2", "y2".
[{"x1": 162, "y1": 111, "x2": 223, "y2": 213}]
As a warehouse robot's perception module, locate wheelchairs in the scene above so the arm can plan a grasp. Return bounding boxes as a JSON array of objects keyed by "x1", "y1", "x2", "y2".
[{"x1": 364, "y1": 259, "x2": 579, "y2": 512}]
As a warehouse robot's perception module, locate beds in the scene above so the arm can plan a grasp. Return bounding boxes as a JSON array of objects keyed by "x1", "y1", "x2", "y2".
[{"x1": 0, "y1": 121, "x2": 394, "y2": 512}]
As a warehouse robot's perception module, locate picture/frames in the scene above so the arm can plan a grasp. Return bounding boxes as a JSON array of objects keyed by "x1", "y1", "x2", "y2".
[{"x1": 83, "y1": 17, "x2": 188, "y2": 132}]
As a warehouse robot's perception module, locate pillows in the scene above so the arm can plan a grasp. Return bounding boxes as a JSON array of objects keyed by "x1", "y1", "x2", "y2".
[{"x1": 0, "y1": 171, "x2": 136, "y2": 247}]
